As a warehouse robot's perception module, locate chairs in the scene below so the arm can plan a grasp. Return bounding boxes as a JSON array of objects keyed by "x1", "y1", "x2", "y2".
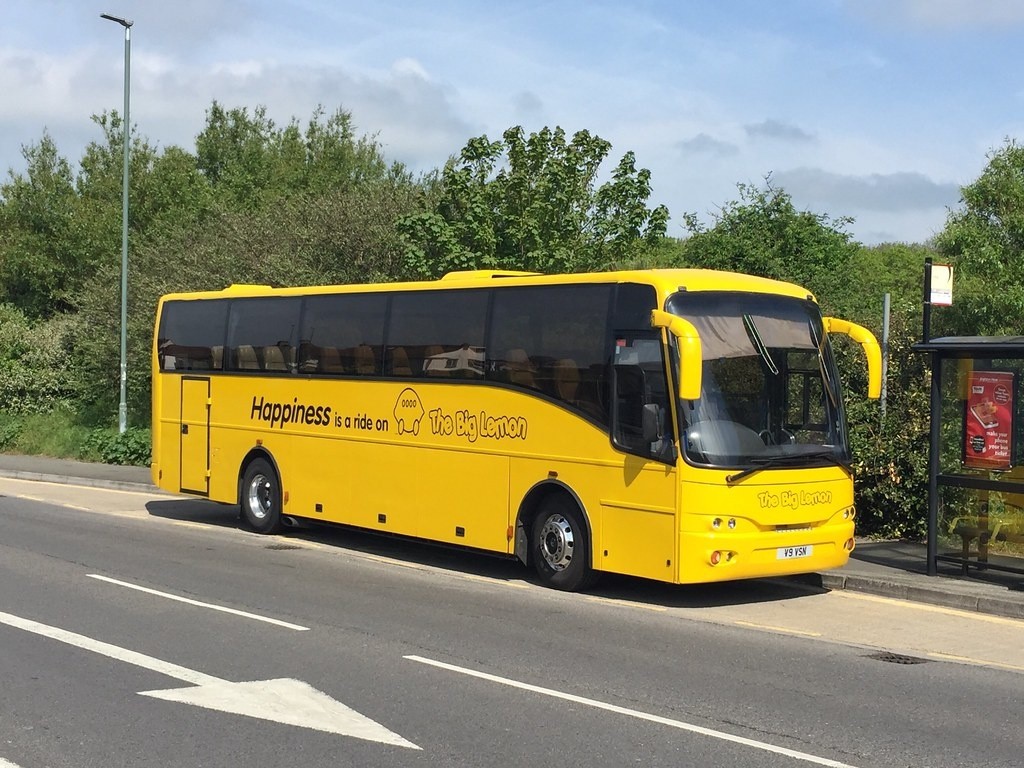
[{"x1": 173, "y1": 335, "x2": 670, "y2": 421}]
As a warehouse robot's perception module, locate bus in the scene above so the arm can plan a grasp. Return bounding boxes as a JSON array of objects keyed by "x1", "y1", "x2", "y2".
[{"x1": 152, "y1": 268, "x2": 886, "y2": 592}]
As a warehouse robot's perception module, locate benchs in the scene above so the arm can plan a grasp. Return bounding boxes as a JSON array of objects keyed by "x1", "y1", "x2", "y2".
[{"x1": 953, "y1": 526, "x2": 1024, "y2": 576}]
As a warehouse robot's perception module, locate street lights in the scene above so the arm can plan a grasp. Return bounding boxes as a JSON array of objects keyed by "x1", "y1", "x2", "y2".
[{"x1": 99, "y1": 14, "x2": 133, "y2": 436}]
